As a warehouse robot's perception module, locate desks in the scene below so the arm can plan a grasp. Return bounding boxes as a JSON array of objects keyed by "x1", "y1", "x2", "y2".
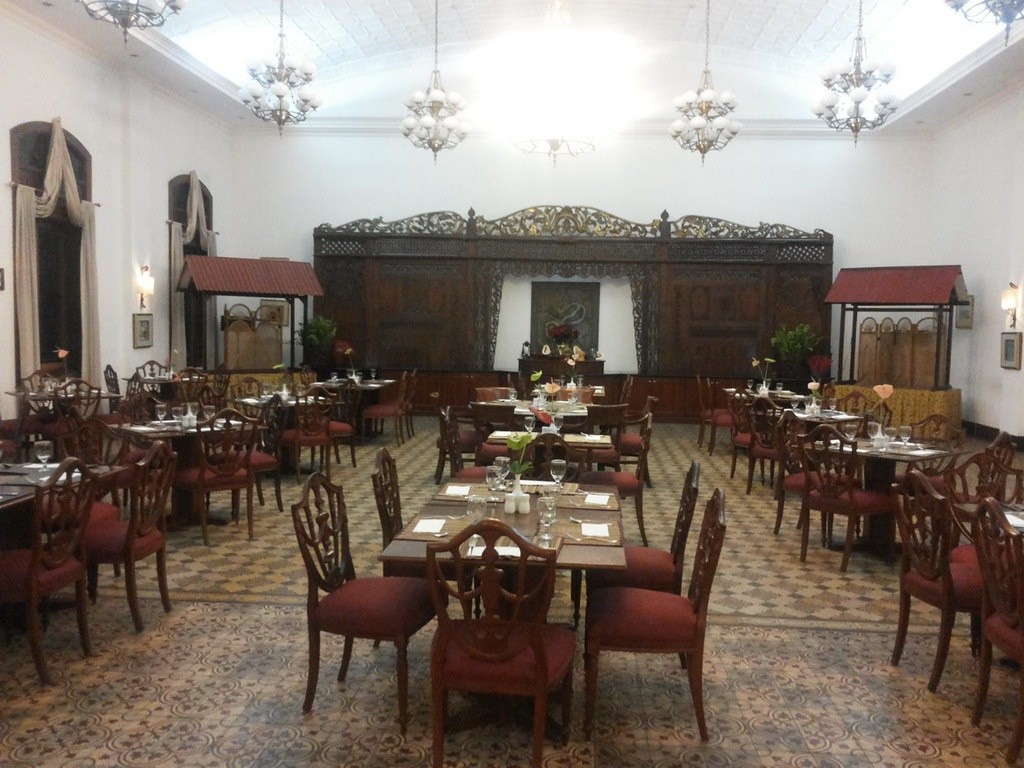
[
  {"x1": 5, "y1": 389, "x2": 124, "y2": 414},
  {"x1": 309, "y1": 377, "x2": 398, "y2": 436},
  {"x1": 791, "y1": 437, "x2": 973, "y2": 559},
  {"x1": 0, "y1": 462, "x2": 129, "y2": 515},
  {"x1": 530, "y1": 381, "x2": 606, "y2": 397},
  {"x1": 730, "y1": 389, "x2": 807, "y2": 407},
  {"x1": 750, "y1": 407, "x2": 864, "y2": 469},
  {"x1": 234, "y1": 394, "x2": 346, "y2": 477},
  {"x1": 953, "y1": 501, "x2": 1024, "y2": 538},
  {"x1": 376, "y1": 477, "x2": 629, "y2": 631},
  {"x1": 104, "y1": 417, "x2": 268, "y2": 523},
  {"x1": 472, "y1": 398, "x2": 601, "y2": 429},
  {"x1": 477, "y1": 430, "x2": 620, "y2": 471}
]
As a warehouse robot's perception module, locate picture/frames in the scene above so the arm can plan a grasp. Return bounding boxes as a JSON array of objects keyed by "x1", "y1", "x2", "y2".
[
  {"x1": 999, "y1": 332, "x2": 1021, "y2": 371},
  {"x1": 535, "y1": 393, "x2": 546, "y2": 408},
  {"x1": 132, "y1": 314, "x2": 154, "y2": 349},
  {"x1": 955, "y1": 295, "x2": 974, "y2": 330}
]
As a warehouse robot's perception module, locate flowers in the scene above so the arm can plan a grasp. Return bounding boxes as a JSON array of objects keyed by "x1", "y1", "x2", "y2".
[
  {"x1": 872, "y1": 383, "x2": 893, "y2": 426},
  {"x1": 53, "y1": 346, "x2": 70, "y2": 378},
  {"x1": 549, "y1": 325, "x2": 580, "y2": 345},
  {"x1": 808, "y1": 377, "x2": 821, "y2": 400},
  {"x1": 752, "y1": 357, "x2": 776, "y2": 382},
  {"x1": 506, "y1": 435, "x2": 535, "y2": 478},
  {"x1": 530, "y1": 369, "x2": 545, "y2": 390},
  {"x1": 272, "y1": 364, "x2": 288, "y2": 384}
]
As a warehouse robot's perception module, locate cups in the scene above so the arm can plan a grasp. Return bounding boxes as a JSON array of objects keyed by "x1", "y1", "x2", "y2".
[
  {"x1": 745, "y1": 379, "x2": 797, "y2": 398},
  {"x1": 42, "y1": 375, "x2": 87, "y2": 397}
]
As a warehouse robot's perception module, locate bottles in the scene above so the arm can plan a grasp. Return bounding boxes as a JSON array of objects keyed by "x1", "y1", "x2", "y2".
[
  {"x1": 505, "y1": 494, "x2": 515, "y2": 514},
  {"x1": 519, "y1": 494, "x2": 530, "y2": 515},
  {"x1": 778, "y1": 390, "x2": 796, "y2": 397}
]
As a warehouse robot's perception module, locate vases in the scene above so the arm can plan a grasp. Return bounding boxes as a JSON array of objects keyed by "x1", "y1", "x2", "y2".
[
  {"x1": 63, "y1": 377, "x2": 72, "y2": 386},
  {"x1": 808, "y1": 397, "x2": 818, "y2": 414},
  {"x1": 184, "y1": 401, "x2": 194, "y2": 423},
  {"x1": 758, "y1": 381, "x2": 770, "y2": 396},
  {"x1": 508, "y1": 474, "x2": 525, "y2": 512},
  {"x1": 558, "y1": 344, "x2": 569, "y2": 356},
  {"x1": 871, "y1": 423, "x2": 888, "y2": 450},
  {"x1": 280, "y1": 383, "x2": 288, "y2": 401}
]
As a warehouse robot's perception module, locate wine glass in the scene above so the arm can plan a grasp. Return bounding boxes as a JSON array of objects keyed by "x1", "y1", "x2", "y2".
[
  {"x1": 506, "y1": 373, "x2": 586, "y2": 413},
  {"x1": 790, "y1": 395, "x2": 837, "y2": 418},
  {"x1": 257, "y1": 367, "x2": 378, "y2": 403},
  {"x1": 150, "y1": 402, "x2": 216, "y2": 430},
  {"x1": 423, "y1": 457, "x2": 619, "y2": 560},
  {"x1": 34, "y1": 441, "x2": 54, "y2": 473},
  {"x1": 844, "y1": 422, "x2": 913, "y2": 454},
  {"x1": 525, "y1": 414, "x2": 564, "y2": 441}
]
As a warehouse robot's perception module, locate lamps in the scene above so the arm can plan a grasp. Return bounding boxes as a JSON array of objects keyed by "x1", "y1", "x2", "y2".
[
  {"x1": 120, "y1": 377, "x2": 217, "y2": 405},
  {"x1": 138, "y1": 266, "x2": 155, "y2": 308},
  {"x1": 1000, "y1": 283, "x2": 1022, "y2": 327}
]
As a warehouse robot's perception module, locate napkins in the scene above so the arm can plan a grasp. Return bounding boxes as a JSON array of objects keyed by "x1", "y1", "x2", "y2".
[
  {"x1": 23, "y1": 462, "x2": 59, "y2": 471},
  {"x1": 910, "y1": 448, "x2": 937, "y2": 455},
  {"x1": 39, "y1": 473, "x2": 81, "y2": 484},
  {"x1": 413, "y1": 518, "x2": 447, "y2": 534},
  {"x1": 836, "y1": 446, "x2": 867, "y2": 453},
  {"x1": 584, "y1": 494, "x2": 610, "y2": 505},
  {"x1": 446, "y1": 485, "x2": 470, "y2": 497},
  {"x1": 580, "y1": 523, "x2": 610, "y2": 539}
]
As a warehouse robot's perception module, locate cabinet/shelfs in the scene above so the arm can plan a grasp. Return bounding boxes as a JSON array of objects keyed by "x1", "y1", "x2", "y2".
[{"x1": 519, "y1": 358, "x2": 605, "y2": 400}]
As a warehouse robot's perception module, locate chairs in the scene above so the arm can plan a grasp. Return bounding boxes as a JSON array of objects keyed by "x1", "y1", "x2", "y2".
[{"x1": 0, "y1": 366, "x2": 1024, "y2": 768}]
